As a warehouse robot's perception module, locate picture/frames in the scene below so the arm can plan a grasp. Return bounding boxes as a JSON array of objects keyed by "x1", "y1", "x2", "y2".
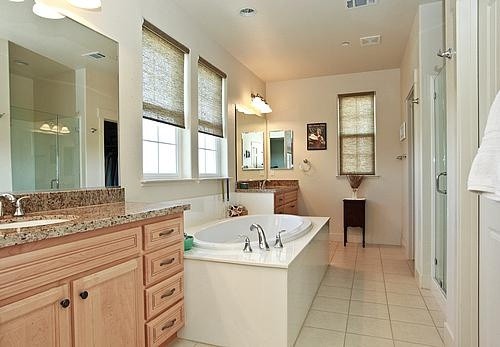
[
  {"x1": 399, "y1": 121, "x2": 406, "y2": 142},
  {"x1": 306, "y1": 122, "x2": 328, "y2": 150}
]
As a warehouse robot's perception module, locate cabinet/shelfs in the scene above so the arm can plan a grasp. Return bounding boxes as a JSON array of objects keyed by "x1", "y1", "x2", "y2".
[
  {"x1": 343, "y1": 198, "x2": 366, "y2": 248},
  {"x1": 142, "y1": 212, "x2": 185, "y2": 347},
  {"x1": 235, "y1": 187, "x2": 298, "y2": 214},
  {"x1": 1, "y1": 220, "x2": 145, "y2": 347}
]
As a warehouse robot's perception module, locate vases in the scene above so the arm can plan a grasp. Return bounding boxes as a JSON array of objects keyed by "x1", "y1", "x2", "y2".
[{"x1": 352, "y1": 187, "x2": 359, "y2": 199}]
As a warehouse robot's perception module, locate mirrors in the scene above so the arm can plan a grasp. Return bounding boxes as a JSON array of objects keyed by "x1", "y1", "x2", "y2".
[
  {"x1": 235, "y1": 105, "x2": 268, "y2": 183},
  {"x1": 269, "y1": 129, "x2": 294, "y2": 170},
  {"x1": 241, "y1": 131, "x2": 265, "y2": 172},
  {"x1": 0, "y1": 1, "x2": 120, "y2": 194}
]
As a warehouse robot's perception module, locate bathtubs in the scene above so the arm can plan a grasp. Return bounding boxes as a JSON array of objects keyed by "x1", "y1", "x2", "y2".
[{"x1": 190, "y1": 214, "x2": 314, "y2": 249}]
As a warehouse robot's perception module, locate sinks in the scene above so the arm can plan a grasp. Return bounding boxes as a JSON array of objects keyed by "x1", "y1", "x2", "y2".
[{"x1": 0, "y1": 219, "x2": 71, "y2": 229}]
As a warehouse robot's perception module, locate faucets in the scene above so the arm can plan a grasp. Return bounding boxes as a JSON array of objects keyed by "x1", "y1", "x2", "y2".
[
  {"x1": 250, "y1": 223, "x2": 269, "y2": 248},
  {"x1": 262, "y1": 179, "x2": 271, "y2": 190},
  {"x1": 0, "y1": 193, "x2": 16, "y2": 216}
]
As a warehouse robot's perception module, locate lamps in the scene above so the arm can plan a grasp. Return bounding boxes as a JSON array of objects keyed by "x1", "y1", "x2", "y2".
[
  {"x1": 250, "y1": 93, "x2": 273, "y2": 116},
  {"x1": 39, "y1": 121, "x2": 71, "y2": 135}
]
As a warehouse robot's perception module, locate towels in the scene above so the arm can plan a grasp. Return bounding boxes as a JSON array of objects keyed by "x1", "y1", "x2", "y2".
[{"x1": 467, "y1": 89, "x2": 500, "y2": 200}]
